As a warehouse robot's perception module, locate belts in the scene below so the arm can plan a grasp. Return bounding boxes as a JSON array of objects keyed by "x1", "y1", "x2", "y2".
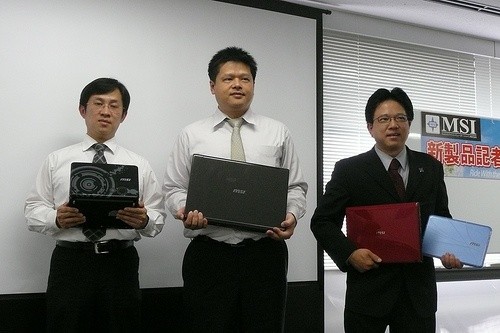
[
  {"x1": 56, "y1": 240, "x2": 134, "y2": 254},
  {"x1": 196, "y1": 234, "x2": 258, "y2": 247}
]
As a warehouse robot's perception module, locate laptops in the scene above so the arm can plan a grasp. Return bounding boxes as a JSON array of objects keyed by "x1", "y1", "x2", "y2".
[
  {"x1": 183, "y1": 153, "x2": 290, "y2": 232},
  {"x1": 66, "y1": 161, "x2": 138, "y2": 230},
  {"x1": 345, "y1": 201, "x2": 424, "y2": 263},
  {"x1": 419, "y1": 215, "x2": 493, "y2": 268}
]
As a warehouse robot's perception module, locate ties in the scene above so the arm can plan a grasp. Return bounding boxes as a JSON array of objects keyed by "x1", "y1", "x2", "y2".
[
  {"x1": 388, "y1": 159, "x2": 406, "y2": 204},
  {"x1": 226, "y1": 118, "x2": 246, "y2": 162},
  {"x1": 82, "y1": 143, "x2": 107, "y2": 242}
]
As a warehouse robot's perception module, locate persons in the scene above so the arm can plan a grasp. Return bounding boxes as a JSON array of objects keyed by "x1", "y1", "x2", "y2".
[
  {"x1": 310, "y1": 86, "x2": 464, "y2": 333},
  {"x1": 22, "y1": 78, "x2": 168, "y2": 333},
  {"x1": 163, "y1": 46, "x2": 308, "y2": 333}
]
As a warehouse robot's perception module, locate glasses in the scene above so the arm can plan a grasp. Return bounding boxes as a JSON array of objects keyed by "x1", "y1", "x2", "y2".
[{"x1": 374, "y1": 114, "x2": 409, "y2": 123}]
